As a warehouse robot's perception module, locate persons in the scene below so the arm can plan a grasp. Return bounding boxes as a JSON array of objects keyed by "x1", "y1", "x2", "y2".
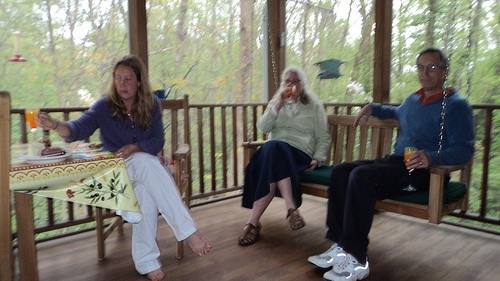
[
  {"x1": 237, "y1": 69, "x2": 333, "y2": 244},
  {"x1": 34, "y1": 57, "x2": 213, "y2": 281},
  {"x1": 308, "y1": 49, "x2": 475, "y2": 281}
]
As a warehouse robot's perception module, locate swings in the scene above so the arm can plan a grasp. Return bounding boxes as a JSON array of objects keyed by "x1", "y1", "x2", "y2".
[{"x1": 241, "y1": 0, "x2": 479, "y2": 226}]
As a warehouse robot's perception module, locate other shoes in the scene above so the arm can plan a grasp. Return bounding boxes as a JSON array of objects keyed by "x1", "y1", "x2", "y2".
[
  {"x1": 285, "y1": 207, "x2": 305, "y2": 230},
  {"x1": 148, "y1": 269, "x2": 164, "y2": 280},
  {"x1": 184, "y1": 239, "x2": 214, "y2": 256},
  {"x1": 238, "y1": 221, "x2": 262, "y2": 246}
]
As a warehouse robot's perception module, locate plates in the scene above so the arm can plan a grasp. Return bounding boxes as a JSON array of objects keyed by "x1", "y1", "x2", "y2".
[
  {"x1": 19, "y1": 149, "x2": 72, "y2": 164},
  {"x1": 66, "y1": 147, "x2": 105, "y2": 157}
]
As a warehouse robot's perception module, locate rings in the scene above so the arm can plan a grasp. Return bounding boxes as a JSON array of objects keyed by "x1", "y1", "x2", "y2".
[{"x1": 419, "y1": 160, "x2": 423, "y2": 165}]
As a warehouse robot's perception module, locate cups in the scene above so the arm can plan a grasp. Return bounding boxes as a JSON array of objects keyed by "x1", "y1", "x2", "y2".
[{"x1": 25, "y1": 106, "x2": 41, "y2": 132}]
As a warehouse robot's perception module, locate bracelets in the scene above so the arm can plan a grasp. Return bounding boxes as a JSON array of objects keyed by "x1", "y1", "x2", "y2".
[{"x1": 52, "y1": 121, "x2": 59, "y2": 130}]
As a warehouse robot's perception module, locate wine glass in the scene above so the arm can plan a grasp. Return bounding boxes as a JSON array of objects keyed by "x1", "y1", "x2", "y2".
[
  {"x1": 402, "y1": 147, "x2": 418, "y2": 192},
  {"x1": 286, "y1": 84, "x2": 297, "y2": 106}
]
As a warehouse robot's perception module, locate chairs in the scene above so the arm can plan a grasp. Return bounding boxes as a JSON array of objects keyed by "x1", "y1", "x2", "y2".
[
  {"x1": 0, "y1": 91, "x2": 16, "y2": 281},
  {"x1": 94, "y1": 93, "x2": 192, "y2": 263}
]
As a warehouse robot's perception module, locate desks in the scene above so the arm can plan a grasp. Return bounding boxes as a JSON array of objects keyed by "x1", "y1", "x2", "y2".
[{"x1": 9, "y1": 141, "x2": 142, "y2": 280}]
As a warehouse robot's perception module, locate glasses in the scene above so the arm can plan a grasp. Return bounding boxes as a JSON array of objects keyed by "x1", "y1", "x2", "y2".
[
  {"x1": 285, "y1": 79, "x2": 302, "y2": 84},
  {"x1": 415, "y1": 63, "x2": 443, "y2": 73}
]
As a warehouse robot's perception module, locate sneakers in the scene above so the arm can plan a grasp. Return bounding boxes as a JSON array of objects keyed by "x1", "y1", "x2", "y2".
[
  {"x1": 322, "y1": 252, "x2": 371, "y2": 281},
  {"x1": 307, "y1": 242, "x2": 348, "y2": 268}
]
